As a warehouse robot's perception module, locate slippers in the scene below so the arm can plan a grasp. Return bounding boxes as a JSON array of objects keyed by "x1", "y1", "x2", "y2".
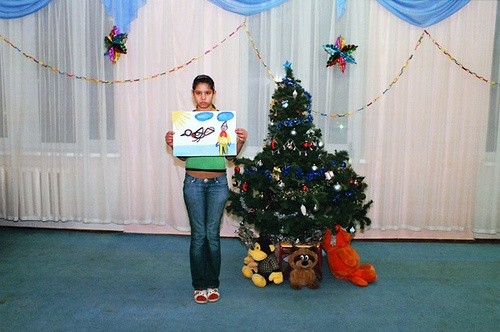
[
  {"x1": 194, "y1": 289, "x2": 208, "y2": 303},
  {"x1": 206, "y1": 288, "x2": 220, "y2": 302}
]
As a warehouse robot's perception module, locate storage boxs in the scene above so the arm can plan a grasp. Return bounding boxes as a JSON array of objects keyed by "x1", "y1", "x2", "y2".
[{"x1": 277, "y1": 242, "x2": 322, "y2": 275}]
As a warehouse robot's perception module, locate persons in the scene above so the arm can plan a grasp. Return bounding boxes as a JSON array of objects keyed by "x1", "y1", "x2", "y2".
[{"x1": 164, "y1": 76, "x2": 250, "y2": 305}]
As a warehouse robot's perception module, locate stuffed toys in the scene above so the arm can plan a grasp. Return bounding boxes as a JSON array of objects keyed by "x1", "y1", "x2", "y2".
[
  {"x1": 241, "y1": 237, "x2": 284, "y2": 289},
  {"x1": 285, "y1": 245, "x2": 323, "y2": 290},
  {"x1": 322, "y1": 224, "x2": 378, "y2": 288}
]
